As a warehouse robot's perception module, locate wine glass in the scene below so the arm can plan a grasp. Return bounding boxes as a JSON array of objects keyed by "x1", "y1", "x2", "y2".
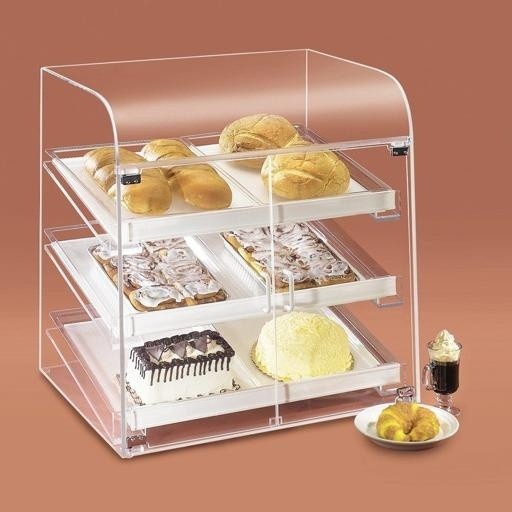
[{"x1": 421, "y1": 339, "x2": 461, "y2": 418}]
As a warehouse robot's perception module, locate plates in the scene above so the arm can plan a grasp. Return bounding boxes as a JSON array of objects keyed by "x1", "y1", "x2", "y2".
[{"x1": 354, "y1": 403, "x2": 459, "y2": 449}]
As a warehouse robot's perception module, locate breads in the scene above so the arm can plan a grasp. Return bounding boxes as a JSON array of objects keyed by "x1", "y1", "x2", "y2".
[
  {"x1": 219, "y1": 114, "x2": 302, "y2": 168},
  {"x1": 93, "y1": 235, "x2": 225, "y2": 311},
  {"x1": 84, "y1": 144, "x2": 172, "y2": 215},
  {"x1": 377, "y1": 402, "x2": 440, "y2": 442},
  {"x1": 139, "y1": 139, "x2": 232, "y2": 210},
  {"x1": 226, "y1": 221, "x2": 359, "y2": 292},
  {"x1": 262, "y1": 139, "x2": 350, "y2": 200}
]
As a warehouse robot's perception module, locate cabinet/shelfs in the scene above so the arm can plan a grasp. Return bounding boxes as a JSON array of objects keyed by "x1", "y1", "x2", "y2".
[{"x1": 39, "y1": 48, "x2": 422, "y2": 460}]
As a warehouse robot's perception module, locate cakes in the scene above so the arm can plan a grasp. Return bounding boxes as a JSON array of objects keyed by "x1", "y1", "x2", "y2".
[
  {"x1": 117, "y1": 328, "x2": 240, "y2": 407},
  {"x1": 256, "y1": 310, "x2": 351, "y2": 380}
]
